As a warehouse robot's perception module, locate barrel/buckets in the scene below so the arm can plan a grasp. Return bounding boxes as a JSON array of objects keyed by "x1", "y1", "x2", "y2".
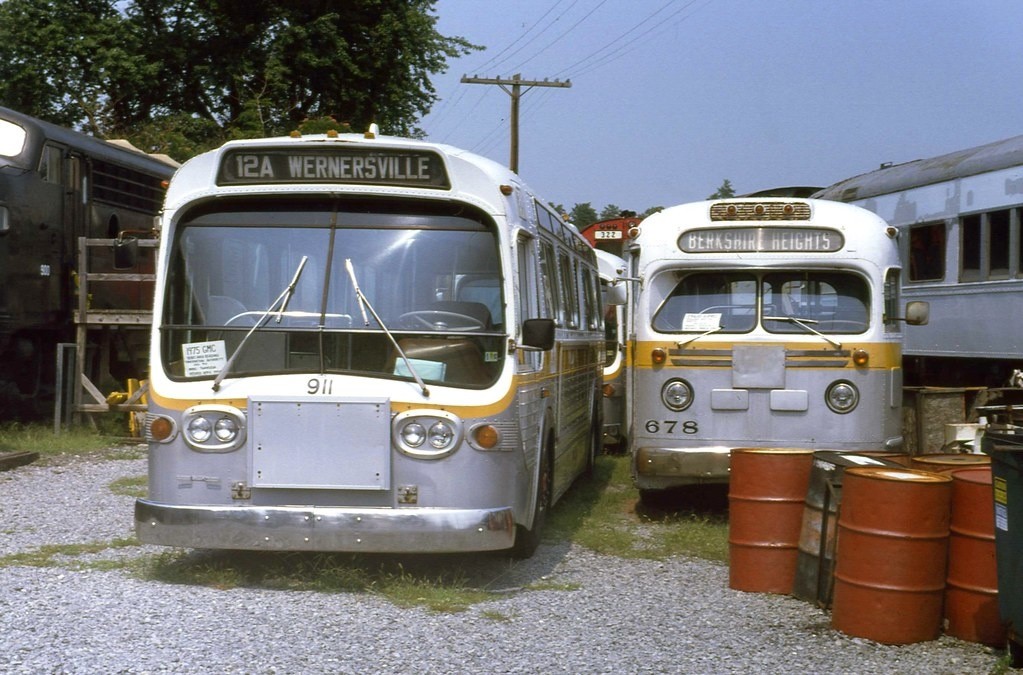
[
  {"x1": 792, "y1": 448, "x2": 906, "y2": 610},
  {"x1": 847, "y1": 451, "x2": 912, "y2": 470},
  {"x1": 943, "y1": 468, "x2": 1007, "y2": 651},
  {"x1": 727, "y1": 448, "x2": 816, "y2": 596},
  {"x1": 912, "y1": 453, "x2": 993, "y2": 475},
  {"x1": 831, "y1": 465, "x2": 954, "y2": 647}
]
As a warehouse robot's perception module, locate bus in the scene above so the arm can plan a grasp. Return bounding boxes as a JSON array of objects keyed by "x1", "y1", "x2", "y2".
[{"x1": 0, "y1": 104, "x2": 1023, "y2": 562}]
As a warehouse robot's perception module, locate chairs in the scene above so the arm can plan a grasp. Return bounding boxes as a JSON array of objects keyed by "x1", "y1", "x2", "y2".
[{"x1": 425, "y1": 301, "x2": 493, "y2": 330}]
